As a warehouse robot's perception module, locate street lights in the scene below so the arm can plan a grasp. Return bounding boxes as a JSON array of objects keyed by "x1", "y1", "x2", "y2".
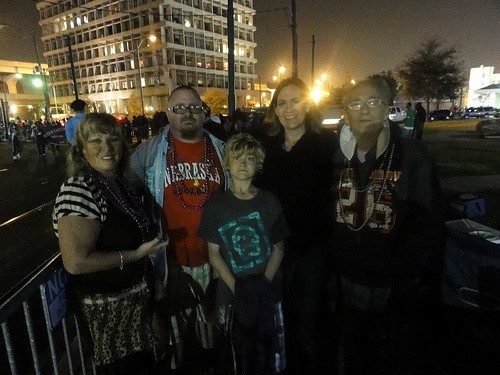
[
  {"x1": 272, "y1": 65, "x2": 286, "y2": 88},
  {"x1": 32, "y1": 79, "x2": 58, "y2": 114},
  {"x1": 13, "y1": 67, "x2": 23, "y2": 80},
  {"x1": 306, "y1": 71, "x2": 331, "y2": 106},
  {"x1": 8, "y1": 103, "x2": 19, "y2": 120},
  {"x1": 137, "y1": 35, "x2": 157, "y2": 116}
]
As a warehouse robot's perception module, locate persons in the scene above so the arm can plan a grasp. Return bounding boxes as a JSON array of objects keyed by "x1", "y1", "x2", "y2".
[{"x1": 0, "y1": 77, "x2": 435, "y2": 375}]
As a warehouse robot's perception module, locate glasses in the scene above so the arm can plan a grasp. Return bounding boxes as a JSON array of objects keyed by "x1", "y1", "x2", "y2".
[
  {"x1": 345, "y1": 98, "x2": 386, "y2": 111},
  {"x1": 167, "y1": 103, "x2": 204, "y2": 115}
]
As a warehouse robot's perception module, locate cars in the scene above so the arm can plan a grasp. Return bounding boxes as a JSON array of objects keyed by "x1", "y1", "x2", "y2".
[
  {"x1": 428, "y1": 110, "x2": 452, "y2": 121},
  {"x1": 460, "y1": 107, "x2": 500, "y2": 118},
  {"x1": 389, "y1": 106, "x2": 407, "y2": 122}
]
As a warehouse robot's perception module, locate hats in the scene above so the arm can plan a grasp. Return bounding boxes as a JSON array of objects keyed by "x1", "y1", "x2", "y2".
[{"x1": 70, "y1": 99, "x2": 87, "y2": 111}]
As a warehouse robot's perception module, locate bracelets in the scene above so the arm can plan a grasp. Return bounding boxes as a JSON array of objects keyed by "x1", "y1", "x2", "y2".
[{"x1": 118, "y1": 249, "x2": 124, "y2": 271}]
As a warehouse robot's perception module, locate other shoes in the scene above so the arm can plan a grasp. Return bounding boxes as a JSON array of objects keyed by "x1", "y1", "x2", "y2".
[
  {"x1": 39, "y1": 153, "x2": 47, "y2": 158},
  {"x1": 12, "y1": 153, "x2": 23, "y2": 160}
]
